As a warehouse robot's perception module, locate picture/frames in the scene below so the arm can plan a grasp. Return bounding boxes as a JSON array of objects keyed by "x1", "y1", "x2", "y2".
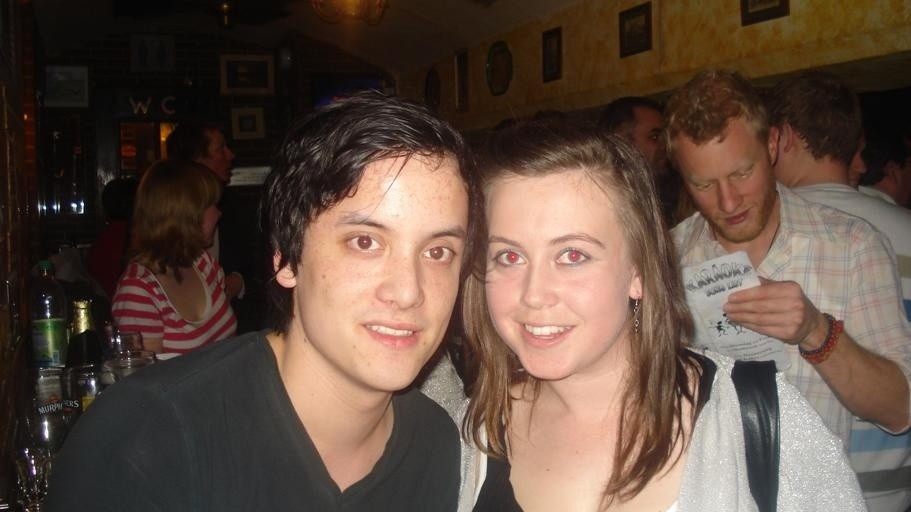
[
  {"x1": 220, "y1": 54, "x2": 275, "y2": 98},
  {"x1": 292, "y1": 62, "x2": 384, "y2": 114},
  {"x1": 39, "y1": 59, "x2": 94, "y2": 112},
  {"x1": 231, "y1": 107, "x2": 263, "y2": 140},
  {"x1": 542, "y1": 26, "x2": 563, "y2": 83},
  {"x1": 484, "y1": 40, "x2": 514, "y2": 96},
  {"x1": 739, "y1": 0, "x2": 789, "y2": 25},
  {"x1": 425, "y1": 70, "x2": 440, "y2": 104},
  {"x1": 619, "y1": 1, "x2": 652, "y2": 59},
  {"x1": 453, "y1": 46, "x2": 470, "y2": 110}
]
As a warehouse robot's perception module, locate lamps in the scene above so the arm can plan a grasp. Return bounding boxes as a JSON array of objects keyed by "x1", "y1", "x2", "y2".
[{"x1": 312, "y1": 0, "x2": 383, "y2": 25}]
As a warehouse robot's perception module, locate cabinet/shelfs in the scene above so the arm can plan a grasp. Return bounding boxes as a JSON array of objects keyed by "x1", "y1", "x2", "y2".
[{"x1": 38, "y1": 126, "x2": 96, "y2": 243}]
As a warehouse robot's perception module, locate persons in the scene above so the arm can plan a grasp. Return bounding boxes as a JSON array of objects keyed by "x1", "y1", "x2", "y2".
[{"x1": 35, "y1": 61, "x2": 911, "y2": 512}]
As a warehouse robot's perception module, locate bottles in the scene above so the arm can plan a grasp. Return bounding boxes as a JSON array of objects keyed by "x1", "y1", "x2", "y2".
[
  {"x1": 31, "y1": 260, "x2": 72, "y2": 368},
  {"x1": 33, "y1": 366, "x2": 66, "y2": 428},
  {"x1": 76, "y1": 361, "x2": 101, "y2": 414},
  {"x1": 65, "y1": 296, "x2": 104, "y2": 397}
]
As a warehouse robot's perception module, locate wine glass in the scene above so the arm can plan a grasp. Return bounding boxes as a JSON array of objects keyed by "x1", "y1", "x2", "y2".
[
  {"x1": 4, "y1": 449, "x2": 53, "y2": 511},
  {"x1": 111, "y1": 351, "x2": 155, "y2": 378},
  {"x1": 103, "y1": 331, "x2": 143, "y2": 359}
]
